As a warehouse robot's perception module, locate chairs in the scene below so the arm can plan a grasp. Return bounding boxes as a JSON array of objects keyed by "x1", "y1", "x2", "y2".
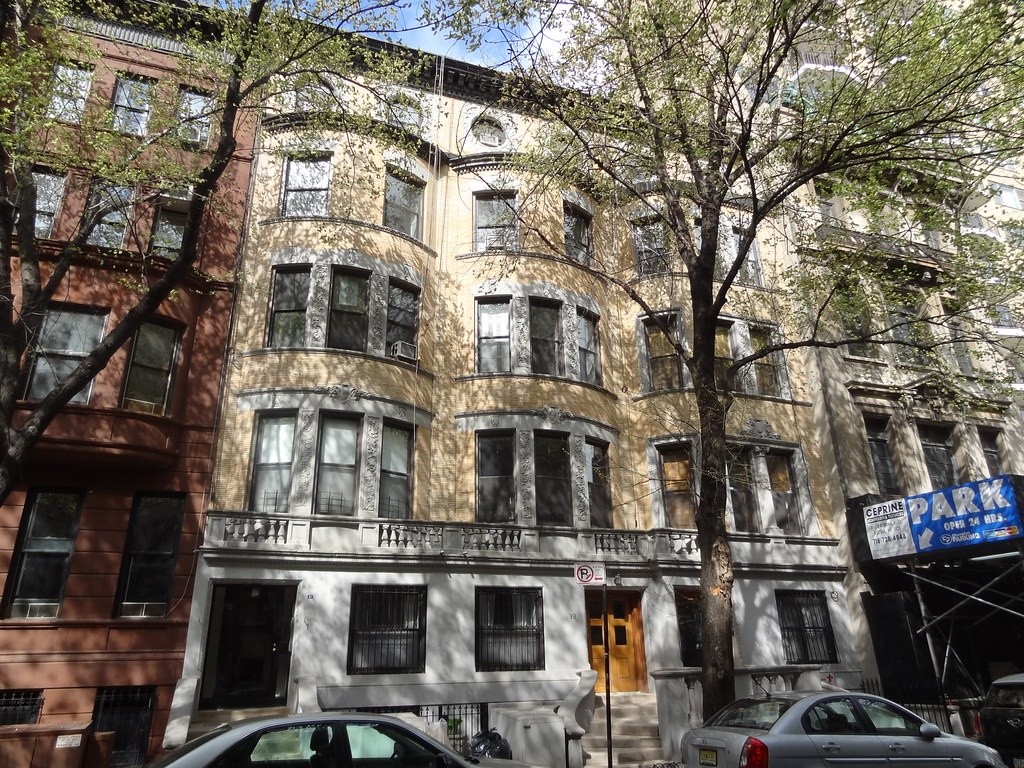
[
  {"x1": 805, "y1": 714, "x2": 818, "y2": 735},
  {"x1": 827, "y1": 715, "x2": 852, "y2": 735},
  {"x1": 309, "y1": 728, "x2": 329, "y2": 768}
]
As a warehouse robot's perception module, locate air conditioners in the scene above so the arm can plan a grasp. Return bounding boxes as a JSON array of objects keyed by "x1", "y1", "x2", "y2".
[
  {"x1": 391, "y1": 340, "x2": 418, "y2": 362},
  {"x1": 485, "y1": 234, "x2": 505, "y2": 250},
  {"x1": 479, "y1": 132, "x2": 498, "y2": 147},
  {"x1": 160, "y1": 180, "x2": 194, "y2": 212},
  {"x1": 177, "y1": 124, "x2": 203, "y2": 148}
]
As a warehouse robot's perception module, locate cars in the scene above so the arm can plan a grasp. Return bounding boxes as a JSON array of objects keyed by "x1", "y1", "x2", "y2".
[
  {"x1": 981, "y1": 673, "x2": 1024, "y2": 759},
  {"x1": 144, "y1": 711, "x2": 540, "y2": 767},
  {"x1": 683, "y1": 691, "x2": 1009, "y2": 767}
]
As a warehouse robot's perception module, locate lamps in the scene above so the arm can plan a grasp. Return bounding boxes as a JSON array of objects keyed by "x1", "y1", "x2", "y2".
[{"x1": 614, "y1": 574, "x2": 624, "y2": 589}]
